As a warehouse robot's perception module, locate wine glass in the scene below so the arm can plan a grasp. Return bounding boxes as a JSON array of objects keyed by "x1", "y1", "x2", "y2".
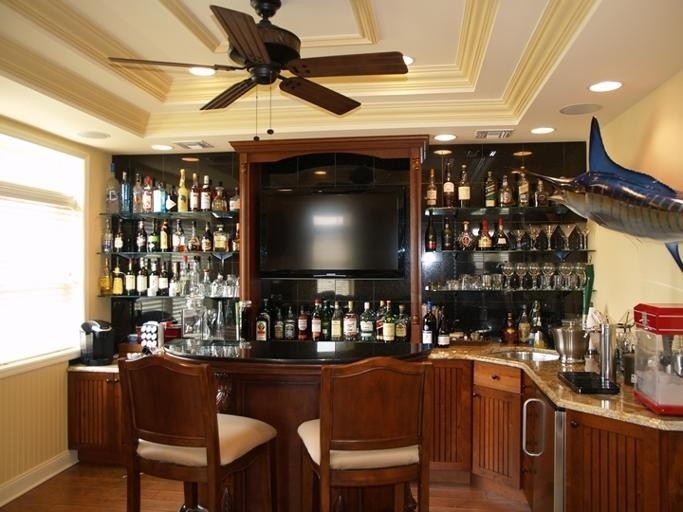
[
  {"x1": 578, "y1": 222, "x2": 593, "y2": 252},
  {"x1": 528, "y1": 263, "x2": 541, "y2": 290},
  {"x1": 542, "y1": 263, "x2": 555, "y2": 290},
  {"x1": 575, "y1": 262, "x2": 587, "y2": 291},
  {"x1": 541, "y1": 224, "x2": 558, "y2": 250},
  {"x1": 511, "y1": 228, "x2": 525, "y2": 251},
  {"x1": 183, "y1": 308, "x2": 217, "y2": 341},
  {"x1": 559, "y1": 263, "x2": 573, "y2": 290},
  {"x1": 560, "y1": 224, "x2": 577, "y2": 251},
  {"x1": 502, "y1": 262, "x2": 514, "y2": 290},
  {"x1": 573, "y1": 222, "x2": 585, "y2": 252},
  {"x1": 515, "y1": 262, "x2": 527, "y2": 290},
  {"x1": 526, "y1": 226, "x2": 540, "y2": 251}
]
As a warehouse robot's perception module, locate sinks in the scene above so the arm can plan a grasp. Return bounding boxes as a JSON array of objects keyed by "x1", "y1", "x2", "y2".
[{"x1": 498, "y1": 351, "x2": 558, "y2": 362}]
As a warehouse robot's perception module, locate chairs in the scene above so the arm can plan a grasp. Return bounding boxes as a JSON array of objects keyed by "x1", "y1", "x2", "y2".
[
  {"x1": 298, "y1": 355, "x2": 430, "y2": 512},
  {"x1": 117, "y1": 354, "x2": 279, "y2": 512}
]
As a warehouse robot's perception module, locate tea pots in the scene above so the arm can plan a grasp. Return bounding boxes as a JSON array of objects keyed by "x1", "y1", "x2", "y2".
[{"x1": 553, "y1": 320, "x2": 591, "y2": 365}]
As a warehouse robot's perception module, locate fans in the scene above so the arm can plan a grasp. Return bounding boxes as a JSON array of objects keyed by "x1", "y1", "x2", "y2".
[{"x1": 106, "y1": 1, "x2": 408, "y2": 115}]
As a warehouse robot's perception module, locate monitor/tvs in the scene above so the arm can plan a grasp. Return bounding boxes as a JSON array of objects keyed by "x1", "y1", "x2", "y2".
[{"x1": 251, "y1": 184, "x2": 409, "y2": 281}]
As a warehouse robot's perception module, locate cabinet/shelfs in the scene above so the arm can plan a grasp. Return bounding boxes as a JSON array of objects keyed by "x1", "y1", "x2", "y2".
[
  {"x1": 131, "y1": 360, "x2": 420, "y2": 488},
  {"x1": 528, "y1": 380, "x2": 562, "y2": 512},
  {"x1": 569, "y1": 407, "x2": 683, "y2": 512},
  {"x1": 422, "y1": 361, "x2": 472, "y2": 477},
  {"x1": 66, "y1": 370, "x2": 133, "y2": 462},
  {"x1": 473, "y1": 358, "x2": 526, "y2": 494}
]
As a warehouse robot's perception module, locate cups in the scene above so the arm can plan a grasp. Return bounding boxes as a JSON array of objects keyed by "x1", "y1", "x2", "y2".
[
  {"x1": 472, "y1": 276, "x2": 482, "y2": 291},
  {"x1": 493, "y1": 274, "x2": 502, "y2": 289},
  {"x1": 483, "y1": 275, "x2": 491, "y2": 288},
  {"x1": 461, "y1": 274, "x2": 472, "y2": 291},
  {"x1": 444, "y1": 279, "x2": 459, "y2": 290}
]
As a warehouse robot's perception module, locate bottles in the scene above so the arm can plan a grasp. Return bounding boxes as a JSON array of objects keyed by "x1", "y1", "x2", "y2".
[
  {"x1": 173, "y1": 261, "x2": 181, "y2": 293},
  {"x1": 297, "y1": 304, "x2": 308, "y2": 339},
  {"x1": 137, "y1": 257, "x2": 148, "y2": 297},
  {"x1": 199, "y1": 271, "x2": 212, "y2": 295},
  {"x1": 121, "y1": 172, "x2": 130, "y2": 213},
  {"x1": 188, "y1": 221, "x2": 200, "y2": 251},
  {"x1": 517, "y1": 166, "x2": 529, "y2": 207},
  {"x1": 283, "y1": 305, "x2": 296, "y2": 339},
  {"x1": 273, "y1": 306, "x2": 284, "y2": 339},
  {"x1": 440, "y1": 216, "x2": 453, "y2": 251},
  {"x1": 99, "y1": 256, "x2": 113, "y2": 295},
  {"x1": 180, "y1": 256, "x2": 191, "y2": 298},
  {"x1": 141, "y1": 176, "x2": 153, "y2": 212},
  {"x1": 477, "y1": 216, "x2": 491, "y2": 250},
  {"x1": 212, "y1": 187, "x2": 227, "y2": 211},
  {"x1": 255, "y1": 314, "x2": 268, "y2": 341},
  {"x1": 160, "y1": 219, "x2": 172, "y2": 251},
  {"x1": 160, "y1": 261, "x2": 169, "y2": 295},
  {"x1": 216, "y1": 301, "x2": 224, "y2": 340},
  {"x1": 421, "y1": 296, "x2": 436, "y2": 352},
  {"x1": 517, "y1": 304, "x2": 531, "y2": 344},
  {"x1": 114, "y1": 219, "x2": 126, "y2": 253},
  {"x1": 103, "y1": 219, "x2": 114, "y2": 253},
  {"x1": 110, "y1": 256, "x2": 124, "y2": 296},
  {"x1": 172, "y1": 219, "x2": 187, "y2": 251},
  {"x1": 157, "y1": 184, "x2": 166, "y2": 213},
  {"x1": 383, "y1": 300, "x2": 396, "y2": 342},
  {"x1": 200, "y1": 221, "x2": 214, "y2": 252},
  {"x1": 309, "y1": 298, "x2": 323, "y2": 340},
  {"x1": 148, "y1": 219, "x2": 159, "y2": 252},
  {"x1": 136, "y1": 221, "x2": 147, "y2": 253},
  {"x1": 426, "y1": 169, "x2": 437, "y2": 207},
  {"x1": 258, "y1": 298, "x2": 274, "y2": 340},
  {"x1": 529, "y1": 300, "x2": 542, "y2": 345},
  {"x1": 438, "y1": 306, "x2": 450, "y2": 346},
  {"x1": 321, "y1": 300, "x2": 332, "y2": 340},
  {"x1": 359, "y1": 301, "x2": 377, "y2": 341},
  {"x1": 484, "y1": 171, "x2": 497, "y2": 207},
  {"x1": 552, "y1": 176, "x2": 568, "y2": 207},
  {"x1": 132, "y1": 173, "x2": 142, "y2": 213},
  {"x1": 169, "y1": 280, "x2": 176, "y2": 297},
  {"x1": 213, "y1": 224, "x2": 229, "y2": 251},
  {"x1": 535, "y1": 180, "x2": 548, "y2": 207},
  {"x1": 376, "y1": 299, "x2": 387, "y2": 340},
  {"x1": 492, "y1": 218, "x2": 509, "y2": 250},
  {"x1": 124, "y1": 255, "x2": 137, "y2": 296},
  {"x1": 197, "y1": 256, "x2": 203, "y2": 280},
  {"x1": 191, "y1": 257, "x2": 200, "y2": 288},
  {"x1": 207, "y1": 256, "x2": 212, "y2": 271},
  {"x1": 229, "y1": 187, "x2": 240, "y2": 212},
  {"x1": 498, "y1": 175, "x2": 512, "y2": 207},
  {"x1": 395, "y1": 304, "x2": 409, "y2": 343},
  {"x1": 177, "y1": 168, "x2": 189, "y2": 212},
  {"x1": 199, "y1": 175, "x2": 214, "y2": 211},
  {"x1": 149, "y1": 261, "x2": 160, "y2": 294},
  {"x1": 189, "y1": 173, "x2": 201, "y2": 211},
  {"x1": 331, "y1": 300, "x2": 343, "y2": 341},
  {"x1": 343, "y1": 300, "x2": 359, "y2": 340},
  {"x1": 459, "y1": 165, "x2": 470, "y2": 208},
  {"x1": 425, "y1": 209, "x2": 436, "y2": 251},
  {"x1": 510, "y1": 214, "x2": 585, "y2": 250},
  {"x1": 443, "y1": 162, "x2": 456, "y2": 207},
  {"x1": 503, "y1": 312, "x2": 517, "y2": 345},
  {"x1": 106, "y1": 162, "x2": 120, "y2": 213},
  {"x1": 456, "y1": 221, "x2": 475, "y2": 251},
  {"x1": 232, "y1": 223, "x2": 240, "y2": 251}
]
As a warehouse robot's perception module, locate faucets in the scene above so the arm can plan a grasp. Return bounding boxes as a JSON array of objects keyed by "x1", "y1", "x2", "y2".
[{"x1": 530, "y1": 325, "x2": 546, "y2": 336}]
